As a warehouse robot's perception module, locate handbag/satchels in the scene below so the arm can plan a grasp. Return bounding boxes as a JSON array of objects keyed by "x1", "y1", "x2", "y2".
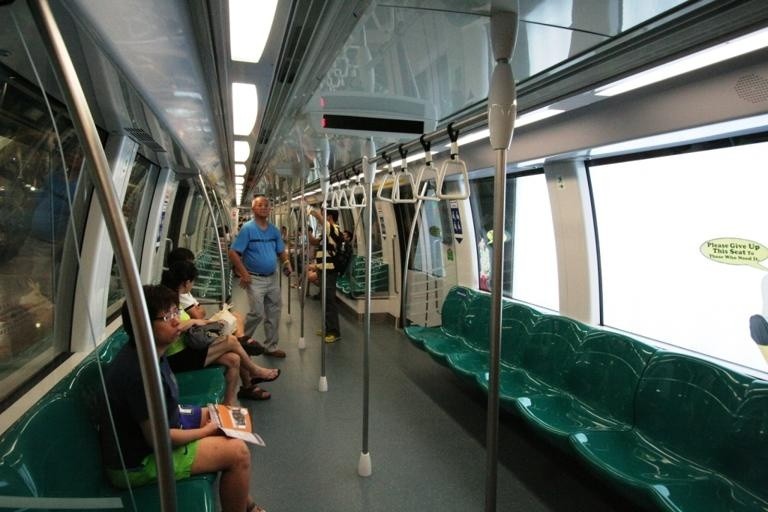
[
  {"x1": 183, "y1": 303, "x2": 238, "y2": 350},
  {"x1": 326, "y1": 228, "x2": 353, "y2": 277}
]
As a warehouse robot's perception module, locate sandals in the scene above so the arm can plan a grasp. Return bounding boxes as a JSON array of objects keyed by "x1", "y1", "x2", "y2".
[{"x1": 237, "y1": 385, "x2": 271, "y2": 401}]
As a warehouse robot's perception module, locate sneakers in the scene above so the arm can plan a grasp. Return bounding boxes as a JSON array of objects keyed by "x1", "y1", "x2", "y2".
[
  {"x1": 315, "y1": 329, "x2": 342, "y2": 343},
  {"x1": 265, "y1": 349, "x2": 286, "y2": 358}
]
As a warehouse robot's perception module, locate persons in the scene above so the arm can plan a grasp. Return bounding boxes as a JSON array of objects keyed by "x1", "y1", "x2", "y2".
[
  {"x1": 160, "y1": 248, "x2": 281, "y2": 409},
  {"x1": 219, "y1": 195, "x2": 353, "y2": 360},
  {"x1": 97, "y1": 284, "x2": 264, "y2": 512}
]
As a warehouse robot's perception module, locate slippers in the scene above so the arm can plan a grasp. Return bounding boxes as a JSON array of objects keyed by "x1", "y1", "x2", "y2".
[{"x1": 250, "y1": 368, "x2": 280, "y2": 385}]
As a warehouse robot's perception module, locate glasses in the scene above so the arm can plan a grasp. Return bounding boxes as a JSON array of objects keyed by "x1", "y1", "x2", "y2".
[{"x1": 152, "y1": 307, "x2": 180, "y2": 323}]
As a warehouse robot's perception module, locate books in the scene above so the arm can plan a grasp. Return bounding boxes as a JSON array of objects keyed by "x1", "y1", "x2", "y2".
[{"x1": 206, "y1": 401, "x2": 266, "y2": 449}]
